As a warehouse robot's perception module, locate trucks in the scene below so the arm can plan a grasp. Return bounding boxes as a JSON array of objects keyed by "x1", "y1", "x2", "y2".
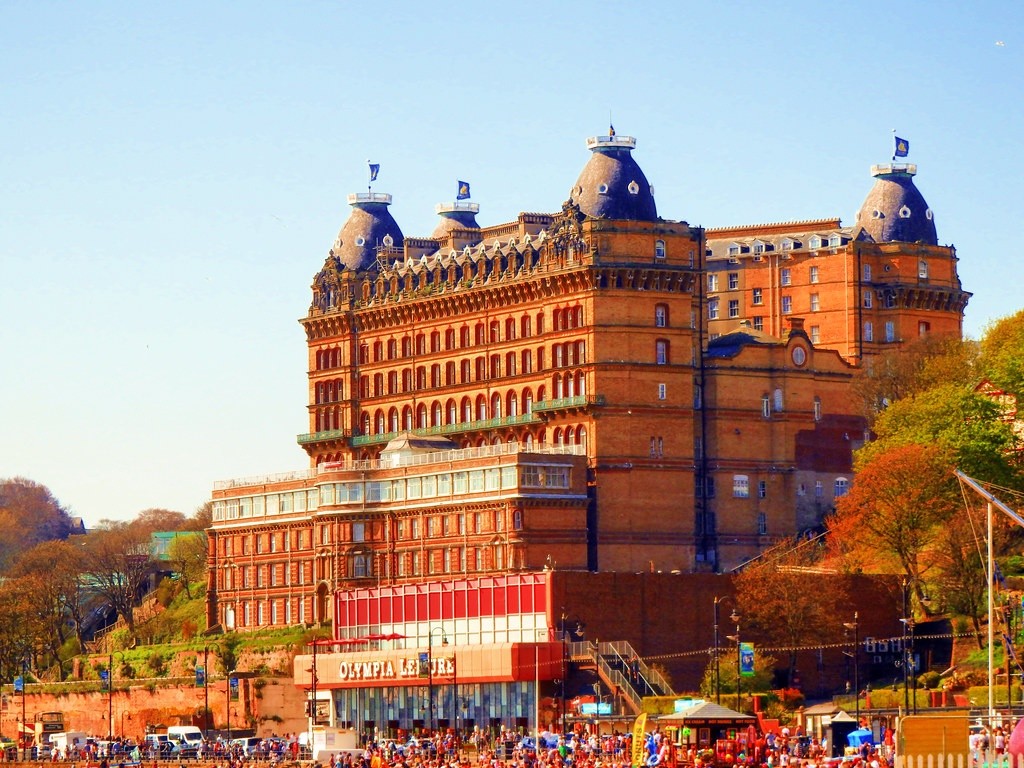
[{"x1": 48, "y1": 733, "x2": 87, "y2": 759}]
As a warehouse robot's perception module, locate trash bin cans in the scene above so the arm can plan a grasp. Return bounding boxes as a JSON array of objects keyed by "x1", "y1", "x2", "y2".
[{"x1": 847, "y1": 729, "x2": 872, "y2": 747}]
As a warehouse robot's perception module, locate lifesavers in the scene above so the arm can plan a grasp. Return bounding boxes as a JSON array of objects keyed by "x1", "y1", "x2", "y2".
[{"x1": 646, "y1": 754, "x2": 660, "y2": 767}]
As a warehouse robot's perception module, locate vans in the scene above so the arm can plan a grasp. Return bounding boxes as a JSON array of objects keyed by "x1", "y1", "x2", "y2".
[
  {"x1": 144, "y1": 726, "x2": 205, "y2": 749},
  {"x1": 96, "y1": 741, "x2": 116, "y2": 758},
  {"x1": 231, "y1": 737, "x2": 261, "y2": 756}
]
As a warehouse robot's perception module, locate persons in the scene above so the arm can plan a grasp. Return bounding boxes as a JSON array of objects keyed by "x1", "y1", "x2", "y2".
[
  {"x1": 329, "y1": 716, "x2": 897, "y2": 768},
  {"x1": 626, "y1": 655, "x2": 639, "y2": 678},
  {"x1": 969, "y1": 723, "x2": 1015, "y2": 765},
  {"x1": 0, "y1": 732, "x2": 312, "y2": 768}
]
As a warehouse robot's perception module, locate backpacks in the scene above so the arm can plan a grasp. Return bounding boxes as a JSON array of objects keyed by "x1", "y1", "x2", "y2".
[{"x1": 982, "y1": 737, "x2": 989, "y2": 747}]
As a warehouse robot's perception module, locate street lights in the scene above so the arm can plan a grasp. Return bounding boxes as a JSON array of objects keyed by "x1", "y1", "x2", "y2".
[
  {"x1": 220, "y1": 664, "x2": 238, "y2": 743},
  {"x1": 713, "y1": 596, "x2": 740, "y2": 706},
  {"x1": 562, "y1": 612, "x2": 585, "y2": 748},
  {"x1": 900, "y1": 577, "x2": 932, "y2": 715},
  {"x1": 108, "y1": 650, "x2": 127, "y2": 755},
  {"x1": 428, "y1": 626, "x2": 449, "y2": 736},
  {"x1": 844, "y1": 610, "x2": 858, "y2": 725},
  {"x1": 204, "y1": 643, "x2": 223, "y2": 739},
  {"x1": 313, "y1": 634, "x2": 333, "y2": 725}
]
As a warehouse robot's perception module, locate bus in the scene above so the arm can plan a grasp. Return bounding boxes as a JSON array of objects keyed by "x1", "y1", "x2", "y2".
[{"x1": 35, "y1": 712, "x2": 65, "y2": 744}]
